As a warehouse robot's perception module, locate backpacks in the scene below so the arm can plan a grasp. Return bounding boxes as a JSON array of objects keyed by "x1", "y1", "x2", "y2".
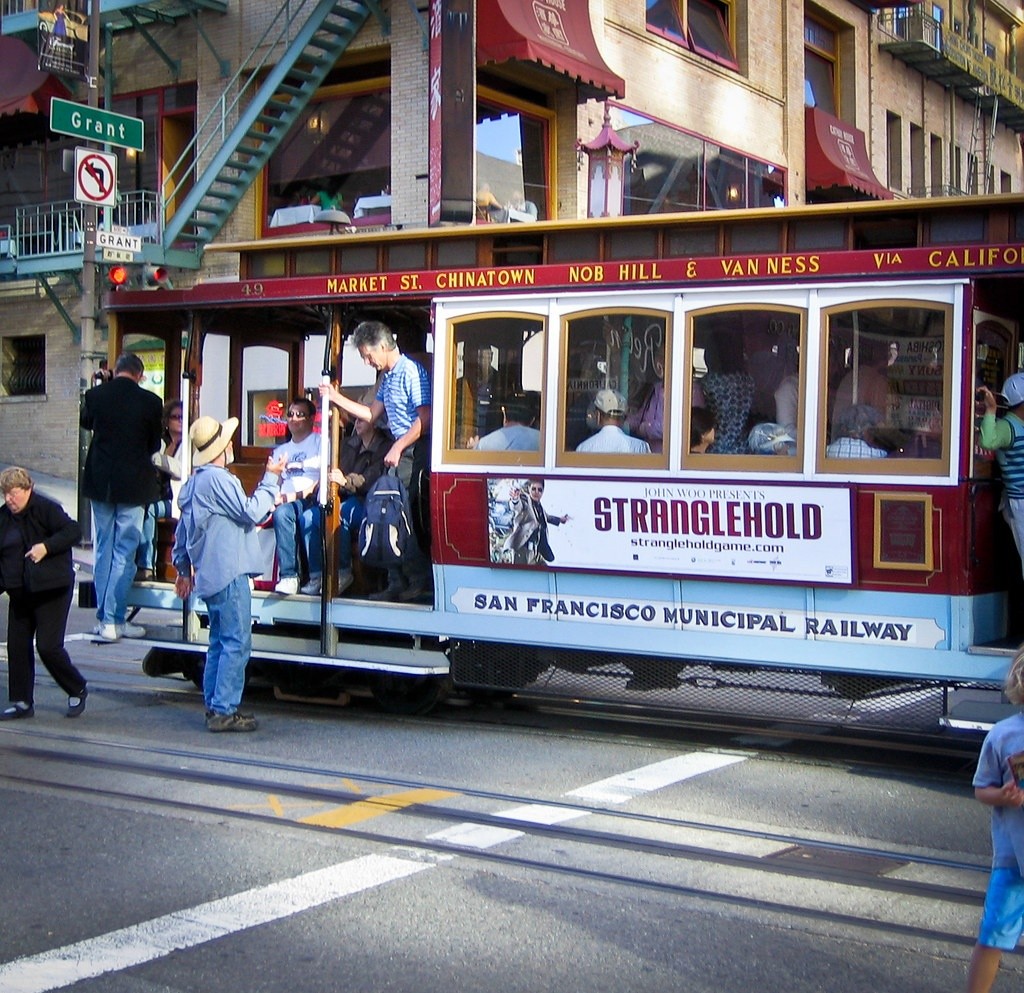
[{"x1": 358, "y1": 466, "x2": 417, "y2": 568}]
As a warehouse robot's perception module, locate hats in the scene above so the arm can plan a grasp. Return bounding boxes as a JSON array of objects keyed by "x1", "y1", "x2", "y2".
[
  {"x1": 594, "y1": 390, "x2": 628, "y2": 416},
  {"x1": 1001, "y1": 373, "x2": 1024, "y2": 406},
  {"x1": 759, "y1": 433, "x2": 797, "y2": 449},
  {"x1": 190, "y1": 416, "x2": 238, "y2": 467}
]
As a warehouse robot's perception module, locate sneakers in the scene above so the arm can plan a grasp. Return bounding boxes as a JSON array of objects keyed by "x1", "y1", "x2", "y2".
[
  {"x1": 94, "y1": 621, "x2": 145, "y2": 640},
  {"x1": 206, "y1": 713, "x2": 258, "y2": 732},
  {"x1": 364, "y1": 571, "x2": 435, "y2": 603},
  {"x1": 302, "y1": 575, "x2": 321, "y2": 595},
  {"x1": 275, "y1": 577, "x2": 301, "y2": 594},
  {"x1": 319, "y1": 571, "x2": 354, "y2": 596}
]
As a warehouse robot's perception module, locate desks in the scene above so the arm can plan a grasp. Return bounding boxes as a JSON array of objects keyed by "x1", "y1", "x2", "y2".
[
  {"x1": 353, "y1": 195, "x2": 392, "y2": 219},
  {"x1": 487, "y1": 208, "x2": 534, "y2": 223},
  {"x1": 270, "y1": 205, "x2": 321, "y2": 228}
]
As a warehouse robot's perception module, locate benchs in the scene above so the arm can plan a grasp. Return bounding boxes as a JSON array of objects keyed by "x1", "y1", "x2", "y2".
[{"x1": 156, "y1": 463, "x2": 266, "y2": 585}]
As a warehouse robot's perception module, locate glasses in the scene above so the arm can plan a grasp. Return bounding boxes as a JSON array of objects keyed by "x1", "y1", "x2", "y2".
[
  {"x1": 353, "y1": 417, "x2": 364, "y2": 422},
  {"x1": 287, "y1": 411, "x2": 309, "y2": 417},
  {"x1": 170, "y1": 414, "x2": 182, "y2": 421},
  {"x1": 713, "y1": 423, "x2": 719, "y2": 430}
]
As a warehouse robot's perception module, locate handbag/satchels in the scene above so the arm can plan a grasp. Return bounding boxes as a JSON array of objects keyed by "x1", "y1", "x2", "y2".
[
  {"x1": 148, "y1": 466, "x2": 173, "y2": 505},
  {"x1": 630, "y1": 429, "x2": 647, "y2": 442},
  {"x1": 249, "y1": 512, "x2": 302, "y2": 591}
]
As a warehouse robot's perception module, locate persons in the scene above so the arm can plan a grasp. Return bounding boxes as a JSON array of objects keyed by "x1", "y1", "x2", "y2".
[
  {"x1": 509, "y1": 475, "x2": 570, "y2": 569},
  {"x1": 0, "y1": 465, "x2": 89, "y2": 720},
  {"x1": 171, "y1": 415, "x2": 289, "y2": 731},
  {"x1": 967, "y1": 648, "x2": 1024, "y2": 993},
  {"x1": 78, "y1": 352, "x2": 164, "y2": 640},
  {"x1": 264, "y1": 398, "x2": 331, "y2": 595},
  {"x1": 321, "y1": 311, "x2": 1024, "y2": 651},
  {"x1": 134, "y1": 400, "x2": 192, "y2": 582}
]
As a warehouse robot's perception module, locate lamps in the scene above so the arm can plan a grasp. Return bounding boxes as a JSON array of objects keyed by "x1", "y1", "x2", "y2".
[{"x1": 307, "y1": 111, "x2": 330, "y2": 144}]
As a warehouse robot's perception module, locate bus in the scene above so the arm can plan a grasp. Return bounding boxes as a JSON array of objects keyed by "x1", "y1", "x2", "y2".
[{"x1": 89, "y1": 189, "x2": 1024, "y2": 709}]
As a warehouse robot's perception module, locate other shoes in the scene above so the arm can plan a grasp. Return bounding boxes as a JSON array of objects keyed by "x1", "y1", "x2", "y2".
[
  {"x1": 67, "y1": 687, "x2": 88, "y2": 718},
  {"x1": 0, "y1": 702, "x2": 34, "y2": 721},
  {"x1": 133, "y1": 570, "x2": 156, "y2": 582}
]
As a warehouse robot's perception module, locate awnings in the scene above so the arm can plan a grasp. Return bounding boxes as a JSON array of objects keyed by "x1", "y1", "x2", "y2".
[
  {"x1": 0, "y1": 36, "x2": 71, "y2": 119},
  {"x1": 476, "y1": 0, "x2": 625, "y2": 104},
  {"x1": 805, "y1": 104, "x2": 894, "y2": 201}
]
